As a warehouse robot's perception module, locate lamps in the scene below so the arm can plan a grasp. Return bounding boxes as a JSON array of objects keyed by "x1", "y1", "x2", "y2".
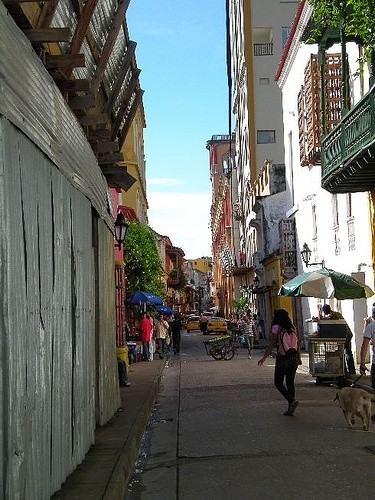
[
  {"x1": 253, "y1": 276, "x2": 260, "y2": 288},
  {"x1": 113, "y1": 212, "x2": 130, "y2": 251},
  {"x1": 301, "y1": 243, "x2": 324, "y2": 269}
]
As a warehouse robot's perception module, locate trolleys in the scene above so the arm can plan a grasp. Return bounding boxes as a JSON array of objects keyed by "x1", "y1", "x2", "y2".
[{"x1": 203, "y1": 334, "x2": 241, "y2": 361}]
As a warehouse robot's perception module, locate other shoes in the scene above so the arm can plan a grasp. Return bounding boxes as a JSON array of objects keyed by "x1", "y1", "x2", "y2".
[
  {"x1": 289, "y1": 400, "x2": 299, "y2": 413},
  {"x1": 120, "y1": 381, "x2": 131, "y2": 387},
  {"x1": 283, "y1": 411, "x2": 294, "y2": 416},
  {"x1": 248, "y1": 356, "x2": 252, "y2": 359}
]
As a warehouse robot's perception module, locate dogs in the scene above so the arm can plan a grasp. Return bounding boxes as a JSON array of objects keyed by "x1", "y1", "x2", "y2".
[{"x1": 331, "y1": 387, "x2": 375, "y2": 431}]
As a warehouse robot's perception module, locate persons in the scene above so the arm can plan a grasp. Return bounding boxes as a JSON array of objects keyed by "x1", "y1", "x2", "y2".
[
  {"x1": 258, "y1": 309, "x2": 303, "y2": 416},
  {"x1": 321, "y1": 302, "x2": 375, "y2": 423},
  {"x1": 140, "y1": 311, "x2": 259, "y2": 362}
]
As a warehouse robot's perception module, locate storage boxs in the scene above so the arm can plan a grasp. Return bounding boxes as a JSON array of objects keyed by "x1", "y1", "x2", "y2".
[
  {"x1": 318, "y1": 320, "x2": 346, "y2": 338},
  {"x1": 127, "y1": 343, "x2": 136, "y2": 354}
]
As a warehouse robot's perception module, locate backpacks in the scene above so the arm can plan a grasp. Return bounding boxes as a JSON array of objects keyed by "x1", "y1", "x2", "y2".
[{"x1": 273, "y1": 324, "x2": 297, "y2": 360}]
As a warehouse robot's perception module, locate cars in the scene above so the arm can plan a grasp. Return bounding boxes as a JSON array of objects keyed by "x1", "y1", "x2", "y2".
[
  {"x1": 207, "y1": 317, "x2": 228, "y2": 335},
  {"x1": 179, "y1": 309, "x2": 214, "y2": 332}
]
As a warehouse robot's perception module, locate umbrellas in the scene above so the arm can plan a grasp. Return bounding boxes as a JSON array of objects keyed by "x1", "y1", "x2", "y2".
[
  {"x1": 279, "y1": 267, "x2": 375, "y2": 318},
  {"x1": 127, "y1": 291, "x2": 171, "y2": 318}
]
